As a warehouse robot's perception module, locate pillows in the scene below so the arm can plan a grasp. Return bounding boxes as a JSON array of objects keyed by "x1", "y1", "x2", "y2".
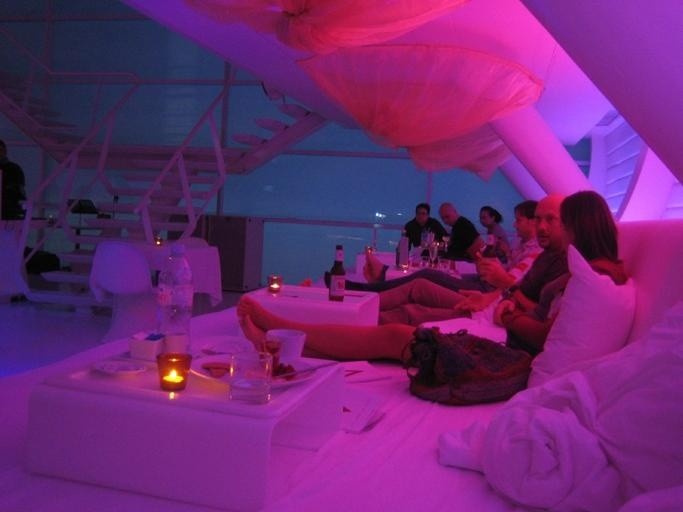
[{"x1": 526, "y1": 244, "x2": 638, "y2": 388}]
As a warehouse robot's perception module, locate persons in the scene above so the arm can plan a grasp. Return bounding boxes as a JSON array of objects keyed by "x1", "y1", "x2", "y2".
[
  {"x1": 235, "y1": 187, "x2": 628, "y2": 364},
  {"x1": 0, "y1": 140, "x2": 26, "y2": 219}
]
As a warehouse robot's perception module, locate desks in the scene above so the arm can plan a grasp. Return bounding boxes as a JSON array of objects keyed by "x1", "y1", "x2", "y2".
[
  {"x1": 22, "y1": 351, "x2": 344, "y2": 512},
  {"x1": 386, "y1": 267, "x2": 461, "y2": 280},
  {"x1": 87, "y1": 238, "x2": 222, "y2": 343},
  {"x1": 239, "y1": 283, "x2": 380, "y2": 332}
]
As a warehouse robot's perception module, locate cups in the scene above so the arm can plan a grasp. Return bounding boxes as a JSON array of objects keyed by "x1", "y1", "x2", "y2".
[
  {"x1": 439, "y1": 258, "x2": 451, "y2": 273},
  {"x1": 155, "y1": 353, "x2": 192, "y2": 392},
  {"x1": 267, "y1": 275, "x2": 282, "y2": 294},
  {"x1": 266, "y1": 329, "x2": 306, "y2": 368},
  {"x1": 228, "y1": 351, "x2": 273, "y2": 405},
  {"x1": 153, "y1": 236, "x2": 163, "y2": 246}
]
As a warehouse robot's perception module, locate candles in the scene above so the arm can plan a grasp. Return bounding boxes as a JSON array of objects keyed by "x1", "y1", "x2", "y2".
[{"x1": 163, "y1": 370, "x2": 184, "y2": 387}]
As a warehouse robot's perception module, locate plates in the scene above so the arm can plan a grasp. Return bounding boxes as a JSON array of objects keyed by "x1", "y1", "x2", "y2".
[
  {"x1": 190, "y1": 354, "x2": 320, "y2": 389},
  {"x1": 94, "y1": 360, "x2": 148, "y2": 378}
]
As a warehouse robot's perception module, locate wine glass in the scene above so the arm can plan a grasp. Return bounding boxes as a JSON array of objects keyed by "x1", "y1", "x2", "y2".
[
  {"x1": 372, "y1": 240, "x2": 377, "y2": 255},
  {"x1": 429, "y1": 246, "x2": 437, "y2": 269}
]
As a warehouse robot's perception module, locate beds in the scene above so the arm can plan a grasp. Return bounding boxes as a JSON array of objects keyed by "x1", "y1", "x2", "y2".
[{"x1": 0, "y1": 220, "x2": 683, "y2": 511}]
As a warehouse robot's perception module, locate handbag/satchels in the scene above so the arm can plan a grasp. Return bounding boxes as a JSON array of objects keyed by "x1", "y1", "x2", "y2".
[{"x1": 400, "y1": 327, "x2": 531, "y2": 406}]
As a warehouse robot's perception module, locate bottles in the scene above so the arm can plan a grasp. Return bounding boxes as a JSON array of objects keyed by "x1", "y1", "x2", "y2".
[
  {"x1": 481, "y1": 230, "x2": 496, "y2": 284},
  {"x1": 399, "y1": 229, "x2": 409, "y2": 272},
  {"x1": 157, "y1": 244, "x2": 194, "y2": 352},
  {"x1": 329, "y1": 245, "x2": 345, "y2": 302}
]
